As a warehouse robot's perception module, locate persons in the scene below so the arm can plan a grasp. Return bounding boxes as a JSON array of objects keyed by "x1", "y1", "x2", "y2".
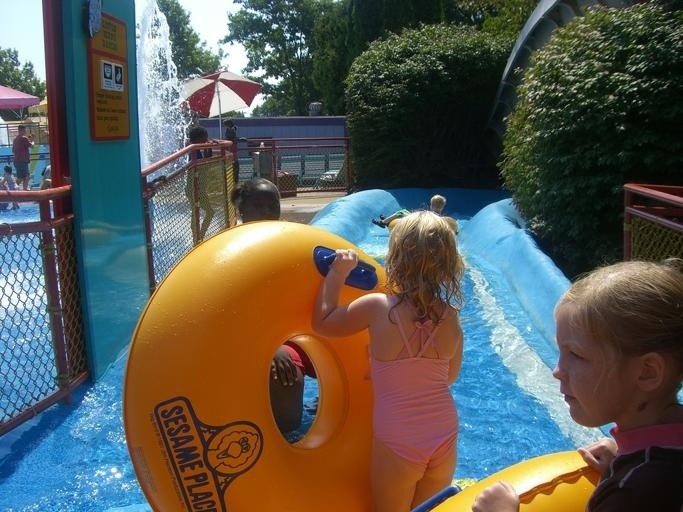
[
  {"x1": 184, "y1": 126, "x2": 219, "y2": 247},
  {"x1": 33, "y1": 164, "x2": 54, "y2": 204},
  {"x1": 311, "y1": 211, "x2": 465, "y2": 512},
  {"x1": 228, "y1": 179, "x2": 317, "y2": 436},
  {"x1": 471, "y1": 254, "x2": 683, "y2": 512},
  {"x1": 11, "y1": 125, "x2": 35, "y2": 191},
  {"x1": 222, "y1": 126, "x2": 247, "y2": 184},
  {"x1": 372, "y1": 195, "x2": 446, "y2": 229},
  {"x1": 0, "y1": 166, "x2": 21, "y2": 210}
]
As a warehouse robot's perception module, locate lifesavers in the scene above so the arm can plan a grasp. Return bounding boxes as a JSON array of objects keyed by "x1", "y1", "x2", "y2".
[
  {"x1": 388, "y1": 214, "x2": 458, "y2": 236},
  {"x1": 124, "y1": 220, "x2": 403, "y2": 512},
  {"x1": 424, "y1": 450, "x2": 603, "y2": 510}
]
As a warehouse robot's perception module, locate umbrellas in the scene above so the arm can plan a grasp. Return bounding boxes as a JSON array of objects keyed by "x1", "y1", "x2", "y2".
[{"x1": 175, "y1": 70, "x2": 264, "y2": 140}]
